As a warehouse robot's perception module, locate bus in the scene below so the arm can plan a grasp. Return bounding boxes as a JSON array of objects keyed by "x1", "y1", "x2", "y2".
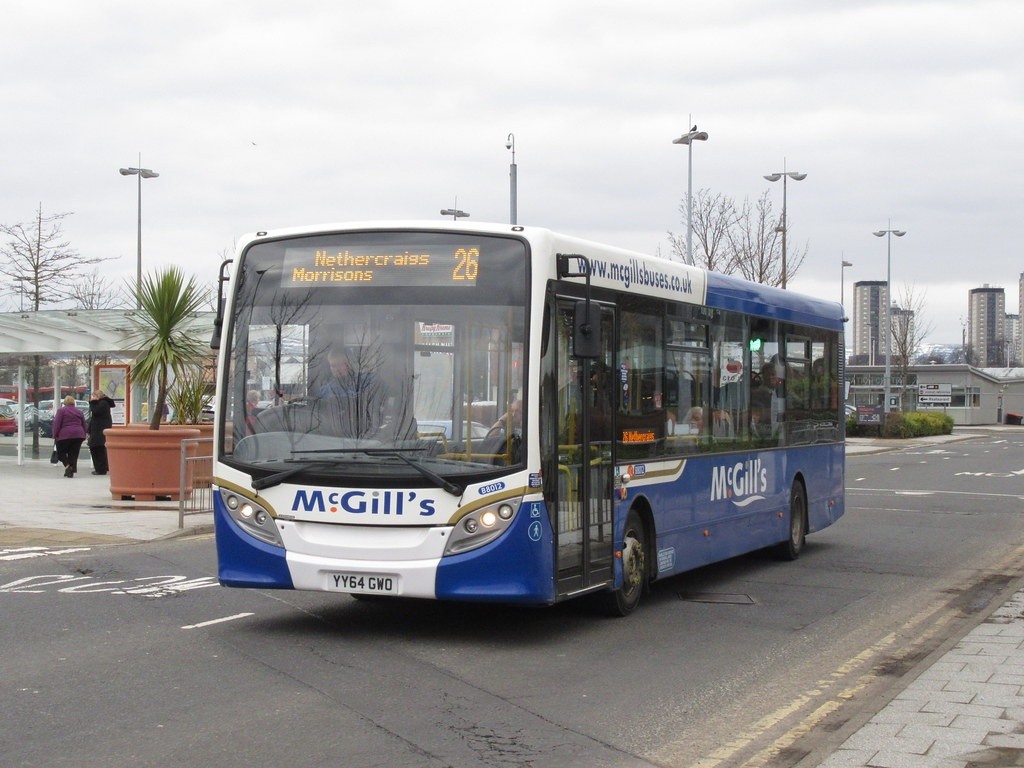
[{"x1": 210, "y1": 219, "x2": 848, "y2": 619}]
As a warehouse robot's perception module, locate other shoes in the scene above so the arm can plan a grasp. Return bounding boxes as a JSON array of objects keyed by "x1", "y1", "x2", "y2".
[
  {"x1": 68, "y1": 473, "x2": 73, "y2": 477},
  {"x1": 64, "y1": 466, "x2": 73, "y2": 476},
  {"x1": 92, "y1": 471, "x2": 106, "y2": 475}
]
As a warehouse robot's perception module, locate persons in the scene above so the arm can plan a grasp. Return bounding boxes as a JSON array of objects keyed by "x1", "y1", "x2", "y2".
[
  {"x1": 51, "y1": 394, "x2": 88, "y2": 476},
  {"x1": 86, "y1": 387, "x2": 114, "y2": 476},
  {"x1": 756, "y1": 362, "x2": 797, "y2": 412},
  {"x1": 487, "y1": 400, "x2": 524, "y2": 441},
  {"x1": 804, "y1": 358, "x2": 838, "y2": 410},
  {"x1": 652, "y1": 392, "x2": 768, "y2": 438},
  {"x1": 316, "y1": 346, "x2": 394, "y2": 414},
  {"x1": 244, "y1": 389, "x2": 261, "y2": 438}
]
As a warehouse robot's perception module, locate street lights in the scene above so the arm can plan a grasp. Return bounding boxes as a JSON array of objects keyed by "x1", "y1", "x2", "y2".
[
  {"x1": 870, "y1": 219, "x2": 906, "y2": 417},
  {"x1": 119, "y1": 151, "x2": 159, "y2": 310},
  {"x1": 671, "y1": 114, "x2": 710, "y2": 262},
  {"x1": 765, "y1": 157, "x2": 808, "y2": 289},
  {"x1": 959, "y1": 319, "x2": 971, "y2": 363},
  {"x1": 503, "y1": 131, "x2": 522, "y2": 224}
]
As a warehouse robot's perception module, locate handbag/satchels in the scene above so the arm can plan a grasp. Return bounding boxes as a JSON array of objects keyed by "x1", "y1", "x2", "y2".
[{"x1": 51, "y1": 440, "x2": 58, "y2": 463}]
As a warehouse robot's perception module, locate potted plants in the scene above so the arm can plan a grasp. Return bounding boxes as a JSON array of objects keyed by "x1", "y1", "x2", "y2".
[
  {"x1": 103, "y1": 264, "x2": 217, "y2": 501},
  {"x1": 128, "y1": 351, "x2": 213, "y2": 489}
]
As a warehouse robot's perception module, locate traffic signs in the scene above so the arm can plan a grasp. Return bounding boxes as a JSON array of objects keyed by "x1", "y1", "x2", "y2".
[
  {"x1": 918, "y1": 395, "x2": 953, "y2": 404},
  {"x1": 918, "y1": 383, "x2": 952, "y2": 395}
]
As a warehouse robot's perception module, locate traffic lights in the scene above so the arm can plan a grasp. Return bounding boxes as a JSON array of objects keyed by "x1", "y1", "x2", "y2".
[{"x1": 747, "y1": 336, "x2": 762, "y2": 352}]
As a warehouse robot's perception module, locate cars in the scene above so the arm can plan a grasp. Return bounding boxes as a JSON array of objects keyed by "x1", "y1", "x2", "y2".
[
  {"x1": 252, "y1": 400, "x2": 271, "y2": 416},
  {"x1": 193, "y1": 393, "x2": 237, "y2": 422},
  {"x1": 844, "y1": 404, "x2": 857, "y2": 419},
  {"x1": 415, "y1": 418, "x2": 493, "y2": 445},
  {"x1": 0, "y1": 394, "x2": 94, "y2": 437}
]
